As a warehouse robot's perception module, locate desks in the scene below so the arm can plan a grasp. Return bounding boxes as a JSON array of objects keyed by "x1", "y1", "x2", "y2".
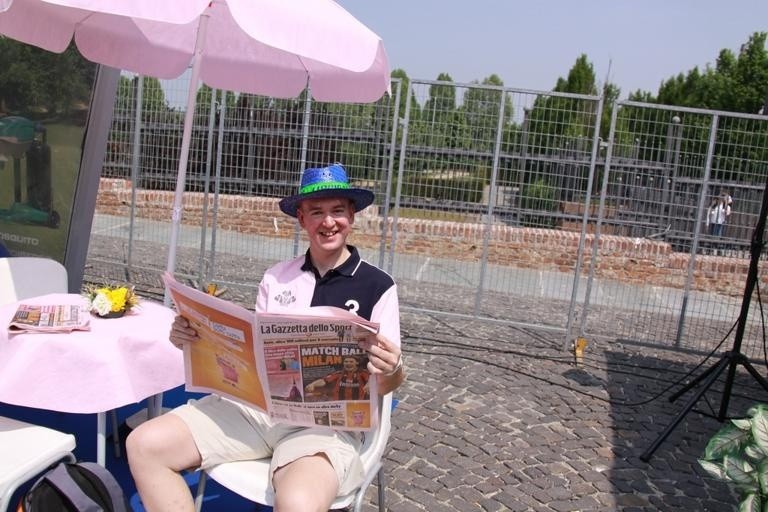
[{"x1": 0, "y1": 294, "x2": 198, "y2": 468}]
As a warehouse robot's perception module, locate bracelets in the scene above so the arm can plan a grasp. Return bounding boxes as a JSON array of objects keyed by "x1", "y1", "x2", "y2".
[{"x1": 383, "y1": 358, "x2": 403, "y2": 377}]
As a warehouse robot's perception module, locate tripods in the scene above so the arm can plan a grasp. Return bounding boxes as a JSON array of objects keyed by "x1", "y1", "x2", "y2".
[{"x1": 640, "y1": 177, "x2": 768, "y2": 463}]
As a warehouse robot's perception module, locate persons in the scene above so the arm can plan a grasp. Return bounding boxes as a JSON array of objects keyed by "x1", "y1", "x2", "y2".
[
  {"x1": 305, "y1": 357, "x2": 369, "y2": 403},
  {"x1": 126, "y1": 163, "x2": 406, "y2": 512},
  {"x1": 704, "y1": 192, "x2": 733, "y2": 256}
]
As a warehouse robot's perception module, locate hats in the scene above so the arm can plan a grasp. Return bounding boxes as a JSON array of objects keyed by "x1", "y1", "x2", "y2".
[{"x1": 278, "y1": 164, "x2": 376, "y2": 218}]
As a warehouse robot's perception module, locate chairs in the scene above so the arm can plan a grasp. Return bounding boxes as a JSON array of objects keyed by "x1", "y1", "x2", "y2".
[
  {"x1": 0, "y1": 256, "x2": 69, "y2": 321},
  {"x1": 194, "y1": 380, "x2": 393, "y2": 512},
  {"x1": 0, "y1": 416, "x2": 76, "y2": 512}
]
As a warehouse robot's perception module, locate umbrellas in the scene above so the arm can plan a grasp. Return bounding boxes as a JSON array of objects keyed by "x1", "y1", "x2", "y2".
[{"x1": 0, "y1": 0, "x2": 393, "y2": 416}]
